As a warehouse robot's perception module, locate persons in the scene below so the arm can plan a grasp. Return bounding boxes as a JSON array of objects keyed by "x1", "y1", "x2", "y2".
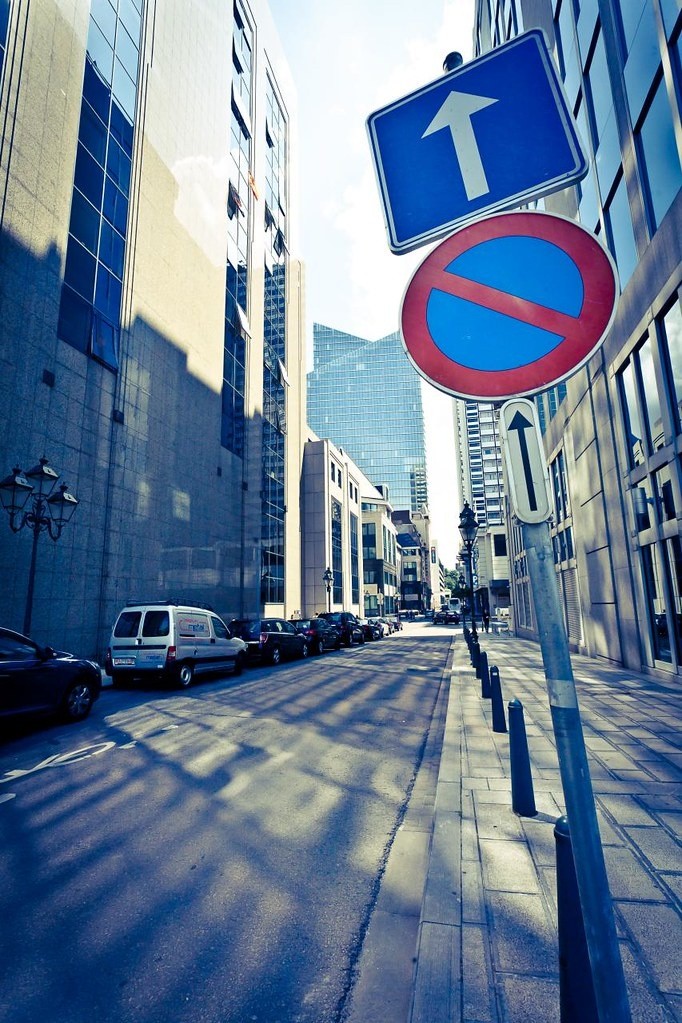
[
  {"x1": 312, "y1": 613, "x2": 319, "y2": 618},
  {"x1": 482, "y1": 609, "x2": 490, "y2": 633},
  {"x1": 406, "y1": 610, "x2": 415, "y2": 620}
]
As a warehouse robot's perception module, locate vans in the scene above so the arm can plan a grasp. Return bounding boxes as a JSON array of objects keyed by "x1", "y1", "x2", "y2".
[{"x1": 105, "y1": 599, "x2": 248, "y2": 690}]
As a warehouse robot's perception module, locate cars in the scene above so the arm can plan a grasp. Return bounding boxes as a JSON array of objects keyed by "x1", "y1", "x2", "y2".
[
  {"x1": 287, "y1": 618, "x2": 342, "y2": 654},
  {"x1": 229, "y1": 617, "x2": 310, "y2": 665},
  {"x1": 356, "y1": 618, "x2": 403, "y2": 640},
  {"x1": 0, "y1": 627, "x2": 102, "y2": 736},
  {"x1": 432, "y1": 610, "x2": 460, "y2": 625}
]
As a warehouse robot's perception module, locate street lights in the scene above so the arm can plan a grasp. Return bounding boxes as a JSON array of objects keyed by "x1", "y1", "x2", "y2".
[
  {"x1": 0, "y1": 454, "x2": 81, "y2": 644},
  {"x1": 322, "y1": 567, "x2": 334, "y2": 613},
  {"x1": 458, "y1": 500, "x2": 481, "y2": 665}
]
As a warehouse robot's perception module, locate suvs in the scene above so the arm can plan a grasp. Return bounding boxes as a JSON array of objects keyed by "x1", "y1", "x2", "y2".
[{"x1": 318, "y1": 611, "x2": 364, "y2": 646}]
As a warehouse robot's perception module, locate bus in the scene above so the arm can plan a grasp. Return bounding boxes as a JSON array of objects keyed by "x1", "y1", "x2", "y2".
[{"x1": 447, "y1": 598, "x2": 461, "y2": 611}]
